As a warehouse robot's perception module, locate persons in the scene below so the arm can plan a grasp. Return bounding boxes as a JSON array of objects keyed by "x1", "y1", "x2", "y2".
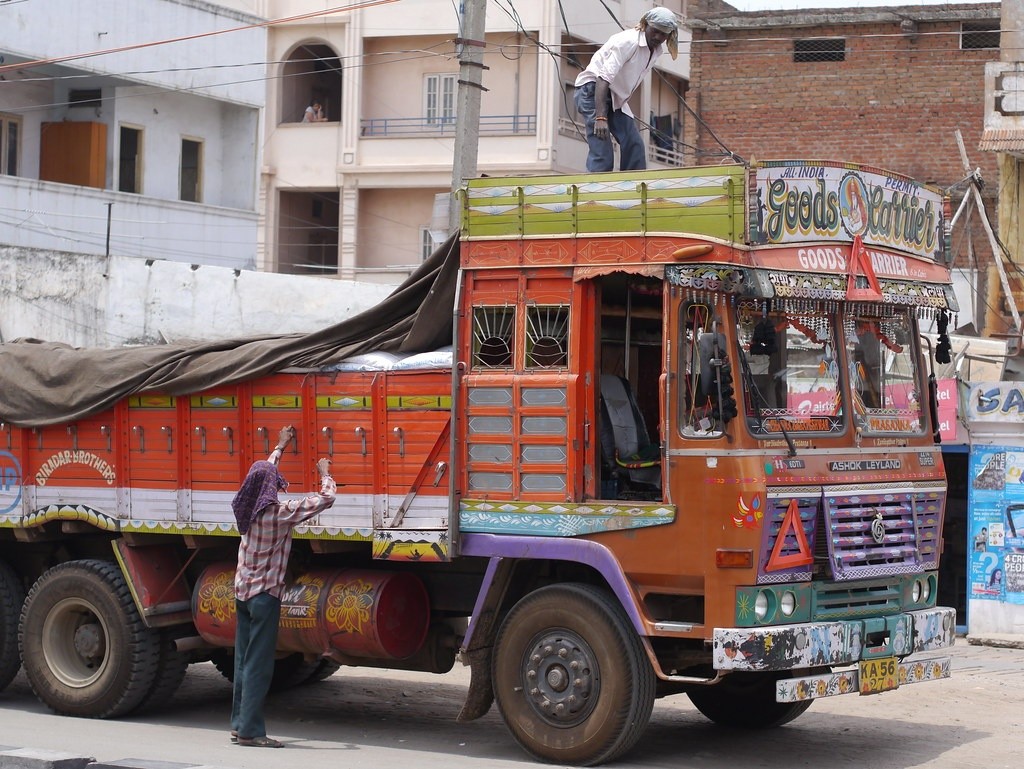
[
  {"x1": 974, "y1": 528, "x2": 987, "y2": 551},
  {"x1": 231, "y1": 424, "x2": 337, "y2": 748},
  {"x1": 990, "y1": 569, "x2": 1002, "y2": 586},
  {"x1": 574, "y1": 7, "x2": 678, "y2": 172},
  {"x1": 302, "y1": 99, "x2": 328, "y2": 122}
]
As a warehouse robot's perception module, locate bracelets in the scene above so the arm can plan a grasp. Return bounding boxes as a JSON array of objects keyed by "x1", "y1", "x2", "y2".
[
  {"x1": 324, "y1": 475, "x2": 332, "y2": 478},
  {"x1": 274, "y1": 446, "x2": 284, "y2": 451},
  {"x1": 596, "y1": 116, "x2": 608, "y2": 121}
]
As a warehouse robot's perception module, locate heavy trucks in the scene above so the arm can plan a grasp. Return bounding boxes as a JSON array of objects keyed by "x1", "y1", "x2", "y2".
[{"x1": 0, "y1": 160, "x2": 961, "y2": 769}]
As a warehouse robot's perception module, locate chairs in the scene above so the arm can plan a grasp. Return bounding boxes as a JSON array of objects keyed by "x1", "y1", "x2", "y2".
[{"x1": 599, "y1": 372, "x2": 661, "y2": 500}]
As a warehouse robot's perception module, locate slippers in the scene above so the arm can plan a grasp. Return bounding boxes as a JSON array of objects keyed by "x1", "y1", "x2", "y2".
[
  {"x1": 238, "y1": 735, "x2": 284, "y2": 748},
  {"x1": 231, "y1": 737, "x2": 238, "y2": 742}
]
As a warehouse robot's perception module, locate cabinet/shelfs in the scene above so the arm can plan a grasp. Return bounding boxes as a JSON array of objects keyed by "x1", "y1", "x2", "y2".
[{"x1": 40, "y1": 122, "x2": 107, "y2": 188}]
